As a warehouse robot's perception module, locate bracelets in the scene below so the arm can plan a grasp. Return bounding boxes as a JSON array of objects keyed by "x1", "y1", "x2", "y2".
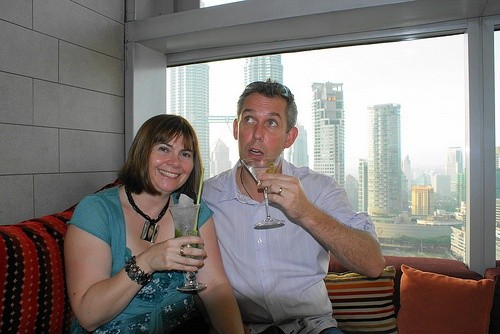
[{"x1": 125, "y1": 255, "x2": 154, "y2": 284}]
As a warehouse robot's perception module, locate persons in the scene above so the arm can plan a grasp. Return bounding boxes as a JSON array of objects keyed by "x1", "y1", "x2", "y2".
[
  {"x1": 66, "y1": 115, "x2": 245, "y2": 334},
  {"x1": 196, "y1": 79, "x2": 385, "y2": 334}
]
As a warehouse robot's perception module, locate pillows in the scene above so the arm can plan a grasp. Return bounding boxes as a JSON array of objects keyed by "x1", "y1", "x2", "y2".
[
  {"x1": 395, "y1": 264, "x2": 495, "y2": 334},
  {"x1": 324, "y1": 266, "x2": 398, "y2": 334}
]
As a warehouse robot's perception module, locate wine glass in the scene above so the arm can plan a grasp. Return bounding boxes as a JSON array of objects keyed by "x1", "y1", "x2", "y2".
[
  {"x1": 241, "y1": 154, "x2": 286, "y2": 226},
  {"x1": 168, "y1": 203, "x2": 207, "y2": 291}
]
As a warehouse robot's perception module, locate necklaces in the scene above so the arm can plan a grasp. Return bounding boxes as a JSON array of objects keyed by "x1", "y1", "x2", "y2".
[
  {"x1": 240, "y1": 167, "x2": 253, "y2": 200},
  {"x1": 124, "y1": 184, "x2": 172, "y2": 243}
]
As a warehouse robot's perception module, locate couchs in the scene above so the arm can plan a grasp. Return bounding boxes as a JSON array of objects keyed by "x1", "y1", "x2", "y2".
[
  {"x1": 328, "y1": 250, "x2": 500, "y2": 334},
  {"x1": 0, "y1": 177, "x2": 133, "y2": 334}
]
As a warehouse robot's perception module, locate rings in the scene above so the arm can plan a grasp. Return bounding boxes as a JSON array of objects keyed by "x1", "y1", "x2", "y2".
[
  {"x1": 180, "y1": 246, "x2": 185, "y2": 256},
  {"x1": 278, "y1": 186, "x2": 284, "y2": 194}
]
